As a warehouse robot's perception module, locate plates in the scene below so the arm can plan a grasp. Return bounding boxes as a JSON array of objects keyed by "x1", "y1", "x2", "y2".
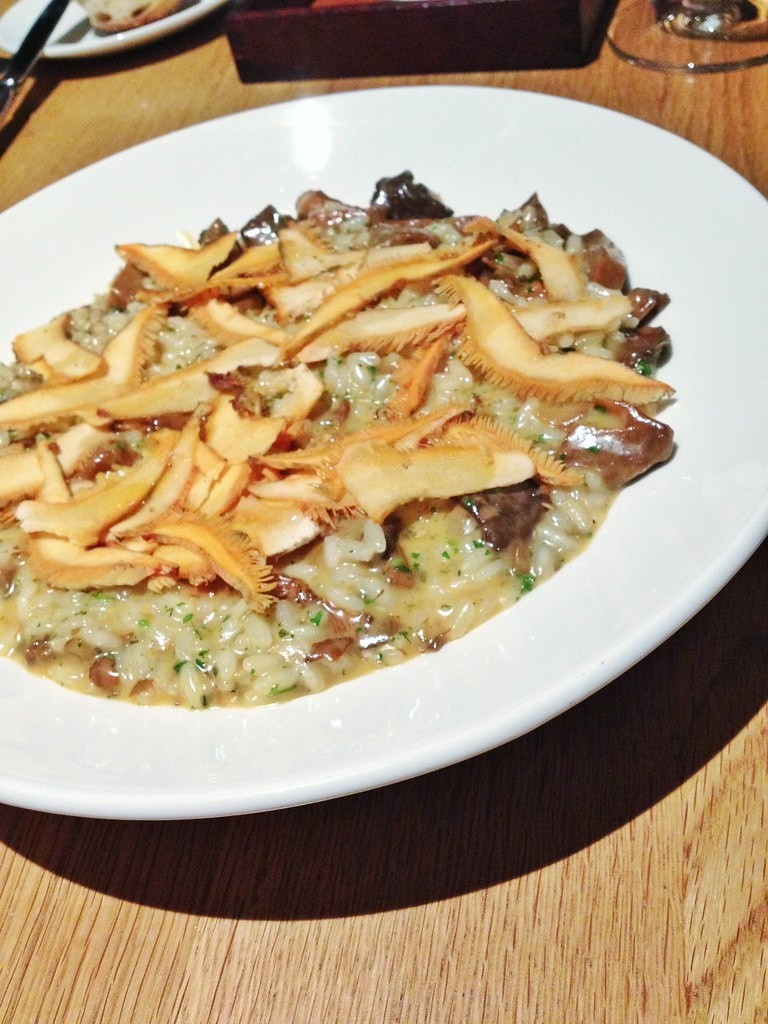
[
  {"x1": 0, "y1": 0, "x2": 226, "y2": 62},
  {"x1": 0, "y1": 84, "x2": 768, "y2": 822}
]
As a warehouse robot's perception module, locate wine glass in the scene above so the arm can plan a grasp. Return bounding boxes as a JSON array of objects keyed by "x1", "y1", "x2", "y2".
[{"x1": 608, "y1": 0, "x2": 768, "y2": 73}]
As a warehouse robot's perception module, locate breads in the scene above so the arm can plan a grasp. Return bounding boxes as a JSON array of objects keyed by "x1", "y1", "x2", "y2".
[{"x1": 76, "y1": 0, "x2": 185, "y2": 32}]
